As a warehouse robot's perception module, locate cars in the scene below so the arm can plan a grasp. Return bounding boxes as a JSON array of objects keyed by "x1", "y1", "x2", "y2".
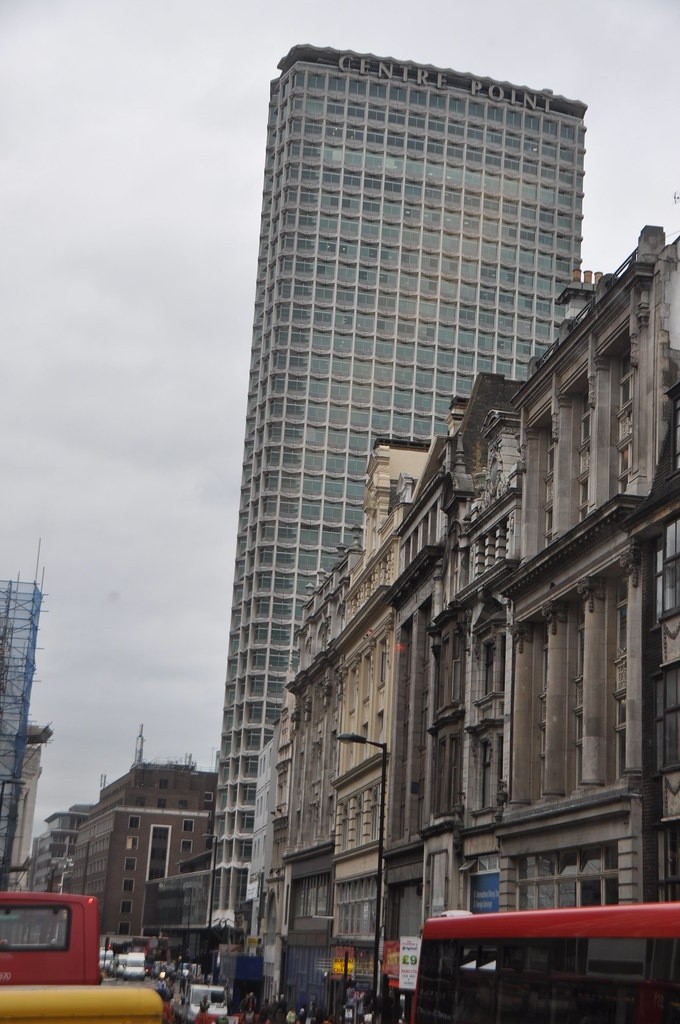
[{"x1": 0, "y1": 888, "x2": 383, "y2": 1024}]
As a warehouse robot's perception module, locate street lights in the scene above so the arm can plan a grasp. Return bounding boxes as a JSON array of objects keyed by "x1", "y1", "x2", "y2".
[{"x1": 336, "y1": 732, "x2": 388, "y2": 1024}]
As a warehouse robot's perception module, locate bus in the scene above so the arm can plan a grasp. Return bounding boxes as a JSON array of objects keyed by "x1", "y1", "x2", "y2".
[{"x1": 410, "y1": 901, "x2": 680, "y2": 1024}]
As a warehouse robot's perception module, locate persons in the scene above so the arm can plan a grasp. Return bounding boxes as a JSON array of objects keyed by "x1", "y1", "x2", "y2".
[{"x1": 97, "y1": 957, "x2": 408, "y2": 1024}]
